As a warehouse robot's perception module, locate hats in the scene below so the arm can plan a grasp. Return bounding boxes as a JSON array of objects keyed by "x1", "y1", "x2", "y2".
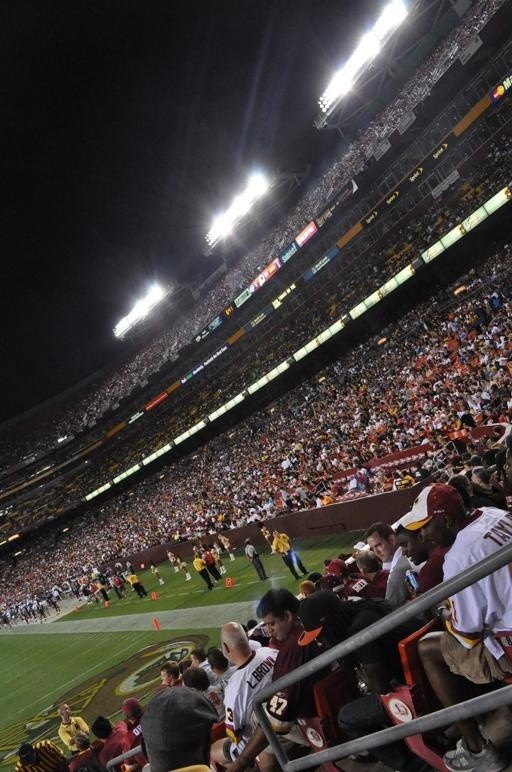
[
  {"x1": 353, "y1": 542, "x2": 365, "y2": 550},
  {"x1": 19, "y1": 743, "x2": 35, "y2": 764},
  {"x1": 296, "y1": 590, "x2": 344, "y2": 647},
  {"x1": 399, "y1": 483, "x2": 464, "y2": 532},
  {"x1": 122, "y1": 698, "x2": 141, "y2": 717},
  {"x1": 139, "y1": 687, "x2": 220, "y2": 746},
  {"x1": 324, "y1": 559, "x2": 348, "y2": 577}
]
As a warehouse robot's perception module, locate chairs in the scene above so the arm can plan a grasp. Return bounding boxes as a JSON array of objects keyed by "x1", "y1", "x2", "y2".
[{"x1": 57, "y1": 563, "x2": 512, "y2": 772}]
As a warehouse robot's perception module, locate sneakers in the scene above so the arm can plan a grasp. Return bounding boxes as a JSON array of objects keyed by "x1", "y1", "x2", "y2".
[{"x1": 441, "y1": 735, "x2": 507, "y2": 771}]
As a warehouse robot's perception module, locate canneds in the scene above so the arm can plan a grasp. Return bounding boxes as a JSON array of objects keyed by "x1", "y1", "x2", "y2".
[{"x1": 405, "y1": 570, "x2": 420, "y2": 592}]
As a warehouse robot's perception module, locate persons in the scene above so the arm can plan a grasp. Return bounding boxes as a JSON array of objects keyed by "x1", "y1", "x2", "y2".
[{"x1": 1, "y1": 1, "x2": 511, "y2": 771}]
